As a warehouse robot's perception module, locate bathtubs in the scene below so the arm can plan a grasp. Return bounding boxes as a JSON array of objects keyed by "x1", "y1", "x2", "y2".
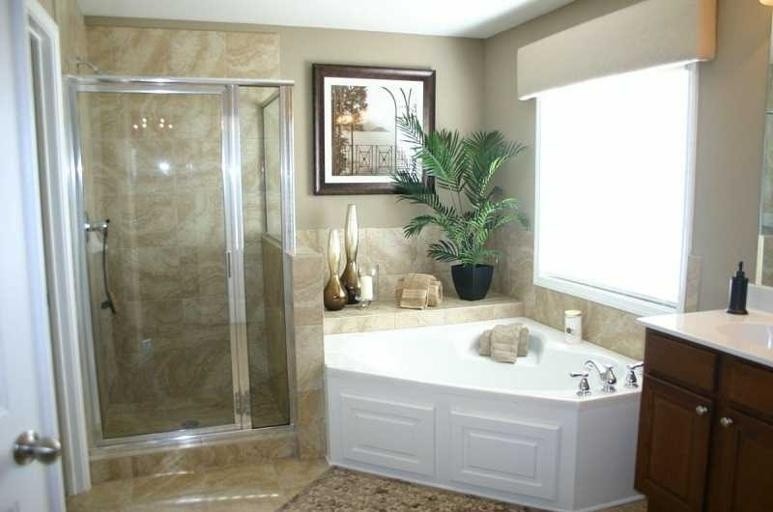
[{"x1": 321, "y1": 316, "x2": 647, "y2": 512}]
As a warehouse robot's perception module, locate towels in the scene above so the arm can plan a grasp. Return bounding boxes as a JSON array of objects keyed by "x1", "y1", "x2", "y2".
[
  {"x1": 394, "y1": 270, "x2": 443, "y2": 309},
  {"x1": 475, "y1": 322, "x2": 532, "y2": 365}
]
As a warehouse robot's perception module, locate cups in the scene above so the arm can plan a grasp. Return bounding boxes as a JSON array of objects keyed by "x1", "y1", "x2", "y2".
[{"x1": 565, "y1": 310, "x2": 582, "y2": 343}]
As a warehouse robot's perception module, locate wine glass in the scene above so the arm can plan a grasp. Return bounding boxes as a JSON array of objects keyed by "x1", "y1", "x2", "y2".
[{"x1": 355, "y1": 262, "x2": 380, "y2": 310}]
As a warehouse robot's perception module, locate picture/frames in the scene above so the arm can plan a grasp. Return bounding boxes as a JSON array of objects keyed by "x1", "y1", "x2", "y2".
[{"x1": 310, "y1": 62, "x2": 437, "y2": 198}]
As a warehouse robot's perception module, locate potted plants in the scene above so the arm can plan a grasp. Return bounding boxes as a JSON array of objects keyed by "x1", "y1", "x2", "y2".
[{"x1": 389, "y1": 108, "x2": 530, "y2": 302}]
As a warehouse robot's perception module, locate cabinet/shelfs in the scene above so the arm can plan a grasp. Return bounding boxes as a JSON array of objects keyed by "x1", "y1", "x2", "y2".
[{"x1": 633, "y1": 328, "x2": 773, "y2": 510}]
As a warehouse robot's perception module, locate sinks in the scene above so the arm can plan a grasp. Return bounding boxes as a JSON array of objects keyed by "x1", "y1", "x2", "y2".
[{"x1": 720, "y1": 322, "x2": 773, "y2": 355}]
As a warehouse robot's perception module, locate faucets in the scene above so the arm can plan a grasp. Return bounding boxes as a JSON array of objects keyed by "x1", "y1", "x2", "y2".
[{"x1": 583, "y1": 360, "x2": 617, "y2": 394}]
